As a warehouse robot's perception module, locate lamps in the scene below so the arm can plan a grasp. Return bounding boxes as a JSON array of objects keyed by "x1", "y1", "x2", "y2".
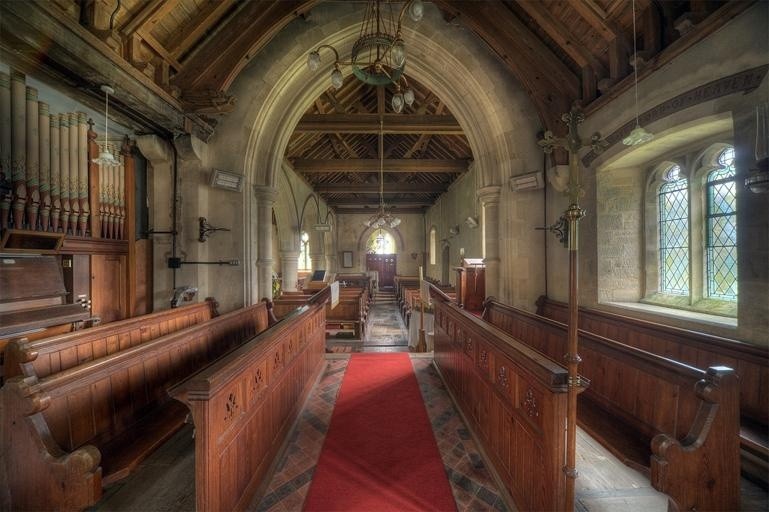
[
  {"x1": 622, "y1": 0, "x2": 655, "y2": 146},
  {"x1": 90, "y1": 85, "x2": 121, "y2": 167},
  {"x1": 306, "y1": 0, "x2": 425, "y2": 114},
  {"x1": 364, "y1": 118, "x2": 401, "y2": 230}
]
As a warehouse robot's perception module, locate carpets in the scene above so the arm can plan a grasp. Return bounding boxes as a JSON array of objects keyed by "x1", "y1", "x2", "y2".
[{"x1": 299, "y1": 352, "x2": 460, "y2": 512}]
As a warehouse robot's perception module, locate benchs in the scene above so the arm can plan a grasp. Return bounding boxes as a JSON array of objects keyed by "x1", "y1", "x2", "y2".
[
  {"x1": 399, "y1": 285, "x2": 456, "y2": 329},
  {"x1": 273, "y1": 288, "x2": 370, "y2": 340}
]
